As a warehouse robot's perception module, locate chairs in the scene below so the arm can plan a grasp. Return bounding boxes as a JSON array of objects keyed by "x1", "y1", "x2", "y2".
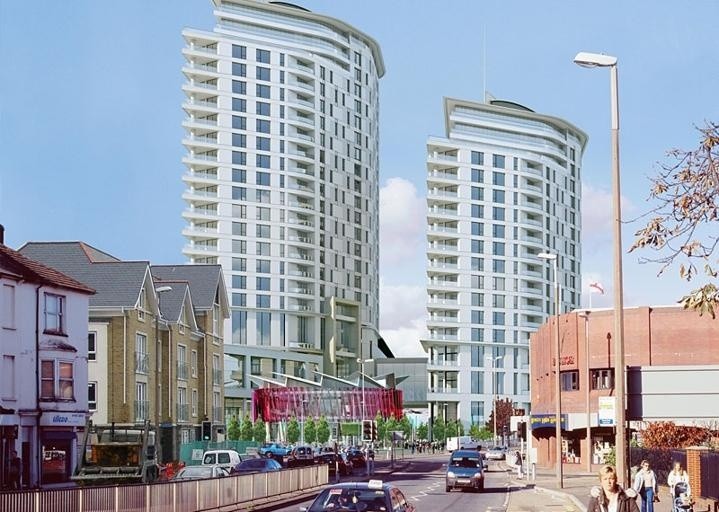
[{"x1": 368, "y1": 498, "x2": 386, "y2": 511}]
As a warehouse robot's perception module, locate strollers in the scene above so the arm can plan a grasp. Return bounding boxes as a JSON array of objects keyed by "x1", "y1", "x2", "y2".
[{"x1": 669, "y1": 480, "x2": 695, "y2": 512}]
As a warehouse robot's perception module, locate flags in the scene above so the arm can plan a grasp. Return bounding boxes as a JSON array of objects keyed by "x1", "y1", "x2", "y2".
[{"x1": 589, "y1": 282, "x2": 607, "y2": 296}]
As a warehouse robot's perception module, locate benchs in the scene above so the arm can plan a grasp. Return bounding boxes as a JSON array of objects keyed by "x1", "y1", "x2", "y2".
[{"x1": 69, "y1": 466, "x2": 143, "y2": 480}]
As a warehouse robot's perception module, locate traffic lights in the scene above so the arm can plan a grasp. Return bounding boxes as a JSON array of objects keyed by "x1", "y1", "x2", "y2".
[
  {"x1": 373, "y1": 422, "x2": 377, "y2": 441},
  {"x1": 503, "y1": 426, "x2": 507, "y2": 432},
  {"x1": 362, "y1": 420, "x2": 371, "y2": 441}
]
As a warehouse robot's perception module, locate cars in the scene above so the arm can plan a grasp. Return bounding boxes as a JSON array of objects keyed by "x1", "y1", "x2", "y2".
[
  {"x1": 299, "y1": 479, "x2": 417, "y2": 512},
  {"x1": 478, "y1": 451, "x2": 489, "y2": 470},
  {"x1": 171, "y1": 439, "x2": 367, "y2": 491},
  {"x1": 485, "y1": 445, "x2": 511, "y2": 459}
]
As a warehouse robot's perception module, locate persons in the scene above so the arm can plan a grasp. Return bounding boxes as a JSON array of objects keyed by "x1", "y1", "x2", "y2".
[
  {"x1": 475, "y1": 445, "x2": 482, "y2": 452},
  {"x1": 7, "y1": 450, "x2": 23, "y2": 490},
  {"x1": 668, "y1": 460, "x2": 692, "y2": 511},
  {"x1": 678, "y1": 491, "x2": 695, "y2": 508},
  {"x1": 634, "y1": 459, "x2": 661, "y2": 511},
  {"x1": 405, "y1": 439, "x2": 436, "y2": 454},
  {"x1": 630, "y1": 462, "x2": 640, "y2": 489},
  {"x1": 514, "y1": 450, "x2": 522, "y2": 480},
  {"x1": 326, "y1": 494, "x2": 353, "y2": 511},
  {"x1": 586, "y1": 464, "x2": 640, "y2": 511}
]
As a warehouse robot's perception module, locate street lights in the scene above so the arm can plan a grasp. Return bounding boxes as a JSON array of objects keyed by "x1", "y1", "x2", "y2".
[
  {"x1": 356, "y1": 355, "x2": 376, "y2": 419},
  {"x1": 570, "y1": 46, "x2": 634, "y2": 511},
  {"x1": 534, "y1": 249, "x2": 567, "y2": 491},
  {"x1": 574, "y1": 308, "x2": 592, "y2": 473},
  {"x1": 487, "y1": 354, "x2": 505, "y2": 444}
]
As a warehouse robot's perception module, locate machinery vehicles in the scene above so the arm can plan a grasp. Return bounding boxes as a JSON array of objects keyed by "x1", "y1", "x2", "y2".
[{"x1": 68, "y1": 418, "x2": 161, "y2": 488}]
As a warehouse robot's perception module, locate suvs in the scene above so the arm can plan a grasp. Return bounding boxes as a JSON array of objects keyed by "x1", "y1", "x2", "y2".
[{"x1": 440, "y1": 449, "x2": 488, "y2": 492}]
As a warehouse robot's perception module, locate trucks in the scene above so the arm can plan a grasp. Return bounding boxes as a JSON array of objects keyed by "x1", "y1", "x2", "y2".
[{"x1": 445, "y1": 435, "x2": 484, "y2": 452}]
